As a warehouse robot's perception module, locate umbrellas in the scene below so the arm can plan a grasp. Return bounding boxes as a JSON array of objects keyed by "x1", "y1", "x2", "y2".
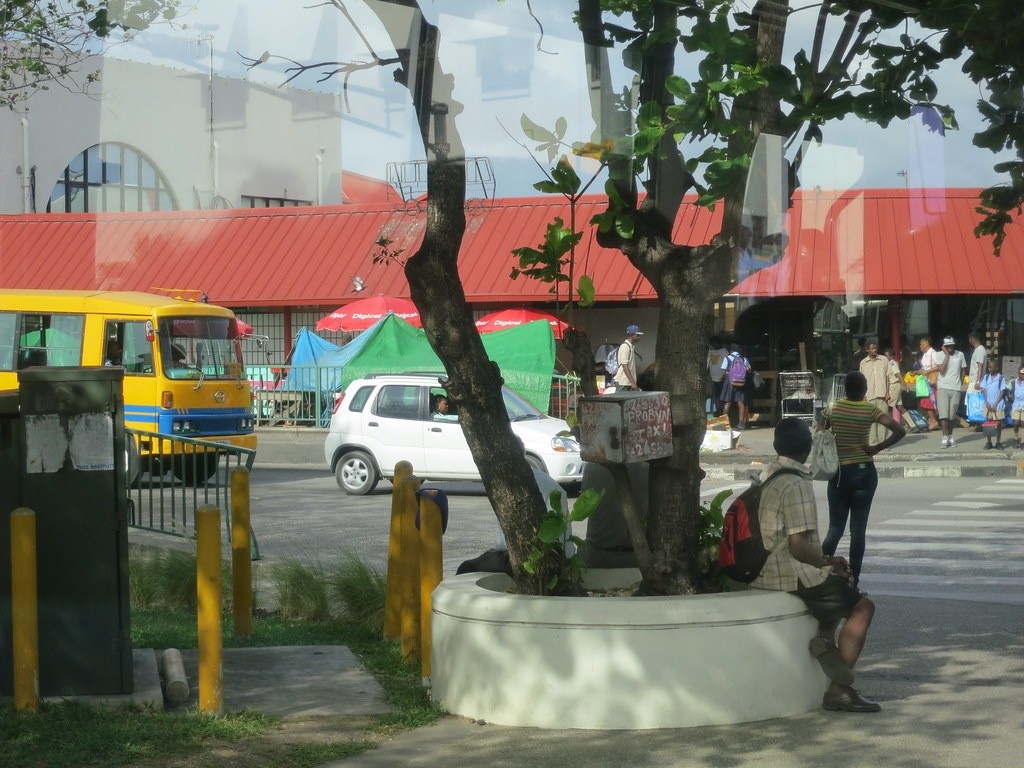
[
  {"x1": 317, "y1": 293, "x2": 567, "y2": 339},
  {"x1": 160, "y1": 307, "x2": 254, "y2": 366}
]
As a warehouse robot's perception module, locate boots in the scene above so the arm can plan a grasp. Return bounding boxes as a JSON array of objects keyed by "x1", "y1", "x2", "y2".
[
  {"x1": 822, "y1": 543, "x2": 836, "y2": 560},
  {"x1": 848, "y1": 571, "x2": 868, "y2": 598}
]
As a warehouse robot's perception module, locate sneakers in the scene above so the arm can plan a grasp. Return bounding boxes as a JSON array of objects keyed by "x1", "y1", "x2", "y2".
[
  {"x1": 984, "y1": 442, "x2": 1004, "y2": 450},
  {"x1": 1013, "y1": 439, "x2": 1024, "y2": 450},
  {"x1": 941, "y1": 435, "x2": 956, "y2": 449}
]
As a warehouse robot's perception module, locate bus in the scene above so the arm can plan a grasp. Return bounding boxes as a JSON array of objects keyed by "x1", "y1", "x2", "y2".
[{"x1": 1, "y1": 289, "x2": 256, "y2": 485}]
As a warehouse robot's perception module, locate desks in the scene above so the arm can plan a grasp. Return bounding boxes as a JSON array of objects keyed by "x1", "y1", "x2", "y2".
[{"x1": 255, "y1": 390, "x2": 302, "y2": 426}]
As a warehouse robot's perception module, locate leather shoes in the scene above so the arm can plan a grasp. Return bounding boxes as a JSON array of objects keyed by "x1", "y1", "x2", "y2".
[
  {"x1": 823, "y1": 687, "x2": 881, "y2": 712},
  {"x1": 809, "y1": 636, "x2": 855, "y2": 685}
]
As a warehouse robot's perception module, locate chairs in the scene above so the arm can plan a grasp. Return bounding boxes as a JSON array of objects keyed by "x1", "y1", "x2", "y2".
[
  {"x1": 107, "y1": 342, "x2": 123, "y2": 358},
  {"x1": 27, "y1": 349, "x2": 47, "y2": 366}
]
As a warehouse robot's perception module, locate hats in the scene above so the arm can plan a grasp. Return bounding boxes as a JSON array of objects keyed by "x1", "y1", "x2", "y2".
[
  {"x1": 627, "y1": 325, "x2": 644, "y2": 334},
  {"x1": 943, "y1": 336, "x2": 955, "y2": 346}
]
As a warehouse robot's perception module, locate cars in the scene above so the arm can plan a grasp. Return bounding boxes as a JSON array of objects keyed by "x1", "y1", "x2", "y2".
[{"x1": 324, "y1": 371, "x2": 583, "y2": 496}]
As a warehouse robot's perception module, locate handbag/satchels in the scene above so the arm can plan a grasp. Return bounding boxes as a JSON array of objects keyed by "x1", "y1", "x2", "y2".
[
  {"x1": 915, "y1": 373, "x2": 929, "y2": 398},
  {"x1": 746, "y1": 365, "x2": 766, "y2": 394},
  {"x1": 802, "y1": 402, "x2": 841, "y2": 488},
  {"x1": 967, "y1": 388, "x2": 987, "y2": 423},
  {"x1": 982, "y1": 410, "x2": 1001, "y2": 436}
]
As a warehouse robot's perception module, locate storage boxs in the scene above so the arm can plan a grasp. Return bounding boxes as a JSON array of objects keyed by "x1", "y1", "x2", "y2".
[{"x1": 982, "y1": 421, "x2": 1001, "y2": 436}]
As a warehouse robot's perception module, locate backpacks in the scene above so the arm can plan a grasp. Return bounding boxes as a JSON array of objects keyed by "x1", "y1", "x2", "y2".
[
  {"x1": 729, "y1": 354, "x2": 747, "y2": 386},
  {"x1": 605, "y1": 342, "x2": 631, "y2": 374},
  {"x1": 907, "y1": 410, "x2": 928, "y2": 433},
  {"x1": 720, "y1": 468, "x2": 802, "y2": 583}
]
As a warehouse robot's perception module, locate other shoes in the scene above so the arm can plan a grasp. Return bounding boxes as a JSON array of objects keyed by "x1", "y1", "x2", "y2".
[
  {"x1": 731, "y1": 421, "x2": 751, "y2": 431},
  {"x1": 927, "y1": 418, "x2": 941, "y2": 431}
]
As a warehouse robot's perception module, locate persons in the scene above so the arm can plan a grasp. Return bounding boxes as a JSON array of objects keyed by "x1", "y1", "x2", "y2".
[
  {"x1": 860, "y1": 332, "x2": 1024, "y2": 453},
  {"x1": 454, "y1": 434, "x2": 574, "y2": 575},
  {"x1": 105, "y1": 341, "x2": 127, "y2": 371},
  {"x1": 853, "y1": 334, "x2": 913, "y2": 371},
  {"x1": 707, "y1": 336, "x2": 752, "y2": 431},
  {"x1": 616, "y1": 325, "x2": 644, "y2": 392},
  {"x1": 814, "y1": 372, "x2": 907, "y2": 596},
  {"x1": 564, "y1": 330, "x2": 600, "y2": 397},
  {"x1": 430, "y1": 394, "x2": 460, "y2": 422},
  {"x1": 749, "y1": 416, "x2": 881, "y2": 712}
]
